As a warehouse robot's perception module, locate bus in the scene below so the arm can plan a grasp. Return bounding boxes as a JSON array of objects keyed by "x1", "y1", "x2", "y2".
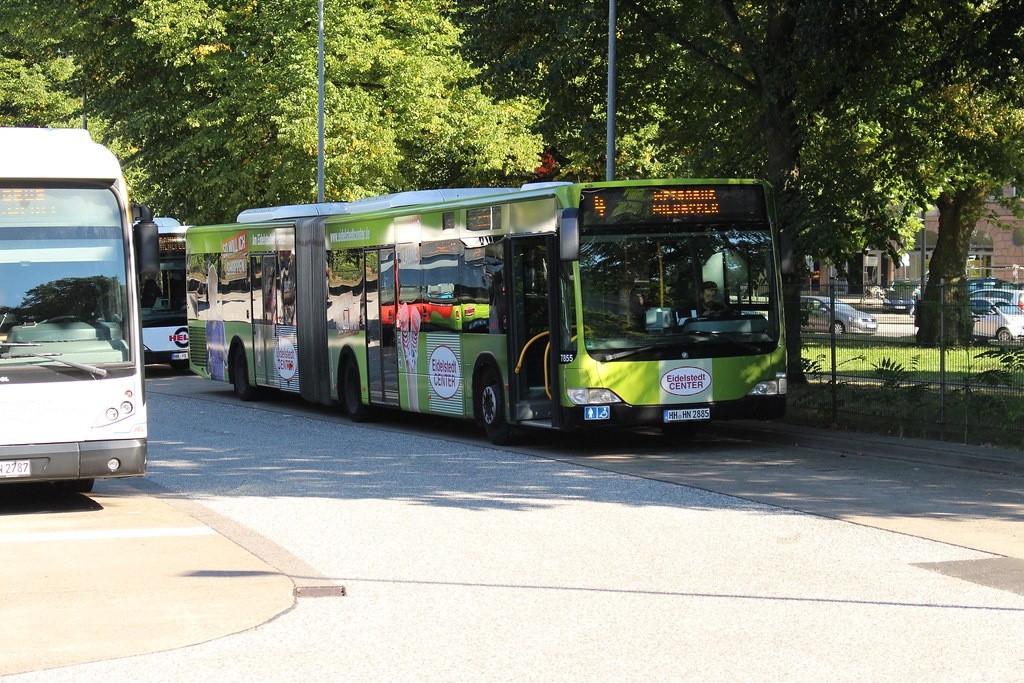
[
  {"x1": 131, "y1": 218, "x2": 198, "y2": 370},
  {"x1": 0, "y1": 124, "x2": 150, "y2": 492},
  {"x1": 185, "y1": 178, "x2": 787, "y2": 447}
]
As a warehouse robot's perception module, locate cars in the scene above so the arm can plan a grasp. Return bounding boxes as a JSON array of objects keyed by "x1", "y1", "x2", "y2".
[
  {"x1": 882, "y1": 279, "x2": 929, "y2": 315},
  {"x1": 970, "y1": 289, "x2": 1024, "y2": 311},
  {"x1": 967, "y1": 277, "x2": 1024, "y2": 291},
  {"x1": 800, "y1": 296, "x2": 878, "y2": 335},
  {"x1": 969, "y1": 297, "x2": 1024, "y2": 343}
]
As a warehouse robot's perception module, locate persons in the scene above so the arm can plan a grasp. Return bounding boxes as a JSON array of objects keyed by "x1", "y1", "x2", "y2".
[
  {"x1": 910, "y1": 284, "x2": 921, "y2": 318},
  {"x1": 687, "y1": 281, "x2": 732, "y2": 321}
]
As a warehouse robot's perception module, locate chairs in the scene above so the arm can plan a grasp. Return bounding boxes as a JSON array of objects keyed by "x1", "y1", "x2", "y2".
[{"x1": 675, "y1": 289, "x2": 696, "y2": 317}]
